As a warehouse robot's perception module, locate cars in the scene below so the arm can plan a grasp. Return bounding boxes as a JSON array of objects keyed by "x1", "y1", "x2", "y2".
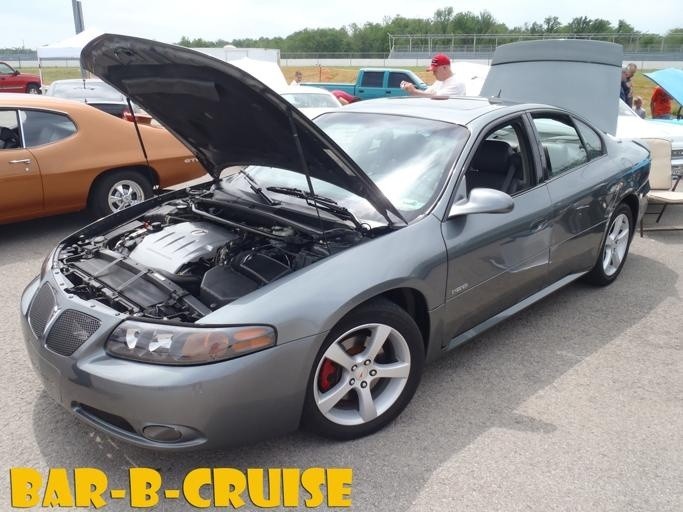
[{"x1": 1, "y1": 33, "x2": 682, "y2": 450}]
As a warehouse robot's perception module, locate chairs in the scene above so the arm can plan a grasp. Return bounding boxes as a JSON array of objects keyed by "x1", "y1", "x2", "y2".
[
  {"x1": 639, "y1": 138, "x2": 683, "y2": 237},
  {"x1": 0, "y1": 127, "x2": 16, "y2": 149},
  {"x1": 465, "y1": 140, "x2": 518, "y2": 196}
]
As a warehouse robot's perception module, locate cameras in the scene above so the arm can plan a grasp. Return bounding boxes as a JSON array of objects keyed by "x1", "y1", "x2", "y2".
[{"x1": 400, "y1": 81, "x2": 405, "y2": 89}]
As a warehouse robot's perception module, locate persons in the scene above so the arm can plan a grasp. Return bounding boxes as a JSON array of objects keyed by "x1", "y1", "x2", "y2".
[
  {"x1": 624, "y1": 63, "x2": 637, "y2": 109},
  {"x1": 314, "y1": 83, "x2": 363, "y2": 107},
  {"x1": 618, "y1": 69, "x2": 632, "y2": 104},
  {"x1": 397, "y1": 50, "x2": 465, "y2": 98},
  {"x1": 649, "y1": 85, "x2": 673, "y2": 122},
  {"x1": 632, "y1": 95, "x2": 646, "y2": 120},
  {"x1": 289, "y1": 69, "x2": 305, "y2": 86}
]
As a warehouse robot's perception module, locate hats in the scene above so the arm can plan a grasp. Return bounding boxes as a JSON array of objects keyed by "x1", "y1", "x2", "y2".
[{"x1": 424, "y1": 54, "x2": 452, "y2": 73}]
